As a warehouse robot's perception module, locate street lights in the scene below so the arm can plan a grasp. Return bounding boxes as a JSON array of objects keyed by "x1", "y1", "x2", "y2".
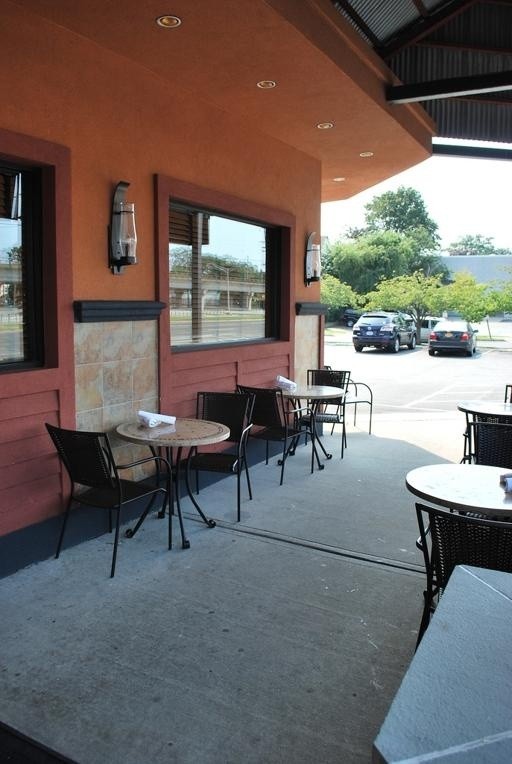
[{"x1": 207, "y1": 263, "x2": 233, "y2": 315}]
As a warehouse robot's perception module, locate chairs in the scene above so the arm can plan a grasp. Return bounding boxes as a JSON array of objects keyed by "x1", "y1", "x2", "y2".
[
  {"x1": 45, "y1": 422, "x2": 174, "y2": 578},
  {"x1": 236, "y1": 383, "x2": 316, "y2": 486},
  {"x1": 296, "y1": 369, "x2": 352, "y2": 460},
  {"x1": 322, "y1": 366, "x2": 374, "y2": 436},
  {"x1": 176, "y1": 391, "x2": 256, "y2": 523},
  {"x1": 402, "y1": 380, "x2": 512, "y2": 654}
]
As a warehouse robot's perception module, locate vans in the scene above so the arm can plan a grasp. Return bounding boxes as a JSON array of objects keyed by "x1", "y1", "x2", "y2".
[{"x1": 349, "y1": 310, "x2": 420, "y2": 354}]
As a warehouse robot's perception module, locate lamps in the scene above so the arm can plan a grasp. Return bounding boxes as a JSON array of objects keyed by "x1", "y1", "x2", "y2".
[
  {"x1": 302, "y1": 230, "x2": 322, "y2": 286},
  {"x1": 107, "y1": 179, "x2": 139, "y2": 274}
]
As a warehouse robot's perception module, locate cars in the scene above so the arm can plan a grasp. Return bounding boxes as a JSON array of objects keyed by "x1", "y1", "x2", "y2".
[
  {"x1": 413, "y1": 316, "x2": 444, "y2": 344},
  {"x1": 342, "y1": 308, "x2": 361, "y2": 327},
  {"x1": 426, "y1": 319, "x2": 480, "y2": 359}
]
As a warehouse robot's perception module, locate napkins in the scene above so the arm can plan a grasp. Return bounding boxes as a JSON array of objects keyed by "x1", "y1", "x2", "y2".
[
  {"x1": 136, "y1": 409, "x2": 177, "y2": 428},
  {"x1": 275, "y1": 376, "x2": 296, "y2": 390}
]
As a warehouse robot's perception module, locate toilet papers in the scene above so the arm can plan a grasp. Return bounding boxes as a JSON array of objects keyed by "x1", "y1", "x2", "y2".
[
  {"x1": 135, "y1": 408, "x2": 176, "y2": 428},
  {"x1": 499, "y1": 473, "x2": 512, "y2": 494},
  {"x1": 274, "y1": 375, "x2": 298, "y2": 390}
]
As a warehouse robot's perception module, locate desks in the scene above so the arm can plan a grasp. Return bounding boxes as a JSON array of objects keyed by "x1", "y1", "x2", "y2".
[
  {"x1": 114, "y1": 417, "x2": 230, "y2": 551},
  {"x1": 273, "y1": 385, "x2": 345, "y2": 471}
]
